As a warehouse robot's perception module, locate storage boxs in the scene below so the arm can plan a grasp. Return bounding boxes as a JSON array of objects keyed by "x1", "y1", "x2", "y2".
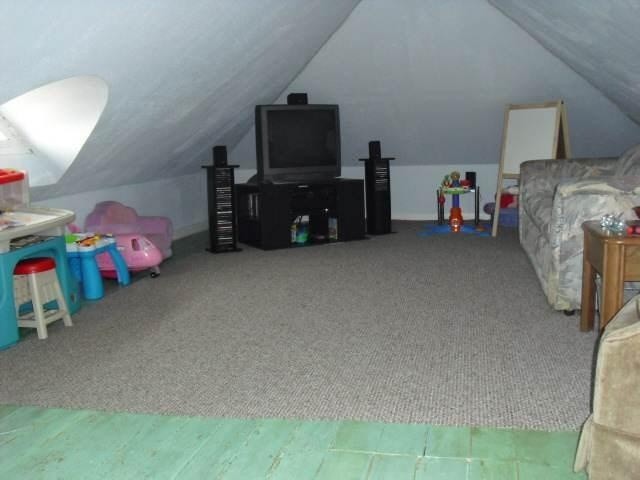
[{"x1": 0, "y1": 169, "x2": 32, "y2": 222}]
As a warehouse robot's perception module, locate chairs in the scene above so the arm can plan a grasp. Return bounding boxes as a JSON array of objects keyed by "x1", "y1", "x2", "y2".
[{"x1": 88, "y1": 198, "x2": 172, "y2": 282}]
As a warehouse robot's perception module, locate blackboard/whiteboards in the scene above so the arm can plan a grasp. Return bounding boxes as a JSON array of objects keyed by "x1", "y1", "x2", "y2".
[{"x1": 499, "y1": 101, "x2": 561, "y2": 179}]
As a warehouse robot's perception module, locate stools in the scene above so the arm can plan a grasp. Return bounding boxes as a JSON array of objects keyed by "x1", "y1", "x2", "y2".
[{"x1": 12, "y1": 234, "x2": 132, "y2": 339}]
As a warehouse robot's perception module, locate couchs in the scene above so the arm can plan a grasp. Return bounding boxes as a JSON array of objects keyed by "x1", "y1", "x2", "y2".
[
  {"x1": 574, "y1": 293, "x2": 640, "y2": 480},
  {"x1": 518, "y1": 147, "x2": 639, "y2": 316}
]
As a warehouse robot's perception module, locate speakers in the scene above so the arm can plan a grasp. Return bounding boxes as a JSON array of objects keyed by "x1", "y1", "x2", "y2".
[
  {"x1": 286, "y1": 92, "x2": 308, "y2": 105},
  {"x1": 212, "y1": 145, "x2": 228, "y2": 165},
  {"x1": 370, "y1": 141, "x2": 381, "y2": 158}
]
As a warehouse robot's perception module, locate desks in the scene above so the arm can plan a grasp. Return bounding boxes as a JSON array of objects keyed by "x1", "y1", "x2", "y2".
[
  {"x1": 0, "y1": 202, "x2": 78, "y2": 350},
  {"x1": 578, "y1": 219, "x2": 640, "y2": 334},
  {"x1": 233, "y1": 175, "x2": 368, "y2": 251}
]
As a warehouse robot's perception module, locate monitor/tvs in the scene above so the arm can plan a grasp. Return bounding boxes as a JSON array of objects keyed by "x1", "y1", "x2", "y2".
[{"x1": 255, "y1": 104, "x2": 342, "y2": 184}]
